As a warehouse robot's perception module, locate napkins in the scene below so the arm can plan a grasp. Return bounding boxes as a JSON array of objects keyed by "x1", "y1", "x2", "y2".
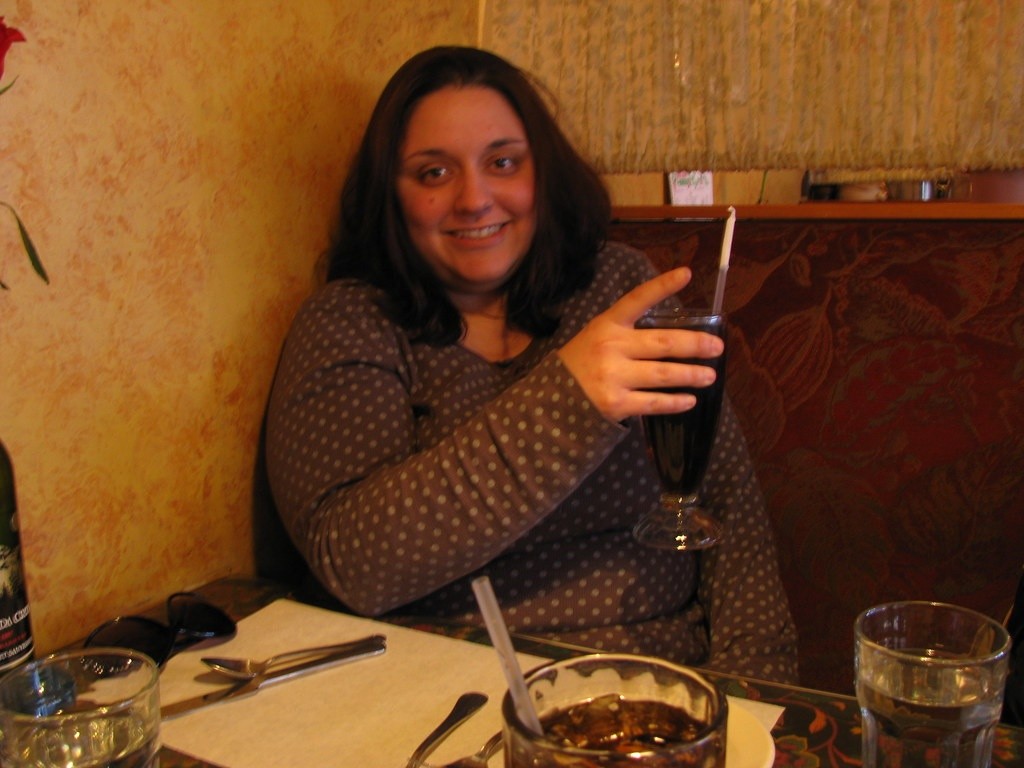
[{"x1": 75, "y1": 597, "x2": 786, "y2": 768}]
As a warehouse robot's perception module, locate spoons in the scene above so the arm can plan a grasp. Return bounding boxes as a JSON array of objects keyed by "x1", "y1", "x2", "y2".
[
  {"x1": 443, "y1": 728, "x2": 502, "y2": 768},
  {"x1": 200, "y1": 635, "x2": 386, "y2": 680}
]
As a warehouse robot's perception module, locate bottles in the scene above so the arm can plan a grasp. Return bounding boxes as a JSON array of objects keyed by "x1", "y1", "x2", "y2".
[{"x1": 0, "y1": 440, "x2": 34, "y2": 678}]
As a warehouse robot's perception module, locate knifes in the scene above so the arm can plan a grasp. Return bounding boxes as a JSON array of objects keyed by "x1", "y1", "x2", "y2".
[{"x1": 159, "y1": 642, "x2": 385, "y2": 722}]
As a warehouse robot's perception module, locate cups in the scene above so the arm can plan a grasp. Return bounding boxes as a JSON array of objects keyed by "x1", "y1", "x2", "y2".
[
  {"x1": 502, "y1": 655, "x2": 729, "y2": 768},
  {"x1": 0, "y1": 648, "x2": 160, "y2": 767},
  {"x1": 855, "y1": 600, "x2": 1012, "y2": 768}
]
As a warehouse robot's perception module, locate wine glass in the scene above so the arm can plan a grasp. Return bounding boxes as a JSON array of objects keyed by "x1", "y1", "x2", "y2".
[{"x1": 631, "y1": 312, "x2": 726, "y2": 553}]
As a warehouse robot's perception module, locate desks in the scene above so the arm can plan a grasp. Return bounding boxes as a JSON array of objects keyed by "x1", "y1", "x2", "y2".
[{"x1": 0, "y1": 571, "x2": 1024, "y2": 768}]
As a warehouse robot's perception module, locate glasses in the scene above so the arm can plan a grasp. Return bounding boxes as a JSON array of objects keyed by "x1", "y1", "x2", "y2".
[{"x1": 82, "y1": 593, "x2": 237, "y2": 677}]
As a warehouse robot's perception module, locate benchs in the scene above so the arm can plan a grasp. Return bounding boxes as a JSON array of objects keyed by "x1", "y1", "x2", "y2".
[{"x1": 596, "y1": 204, "x2": 1024, "y2": 719}]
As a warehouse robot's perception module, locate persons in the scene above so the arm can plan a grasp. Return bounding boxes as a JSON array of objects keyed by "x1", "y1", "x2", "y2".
[{"x1": 263, "y1": 46, "x2": 797, "y2": 685}]
